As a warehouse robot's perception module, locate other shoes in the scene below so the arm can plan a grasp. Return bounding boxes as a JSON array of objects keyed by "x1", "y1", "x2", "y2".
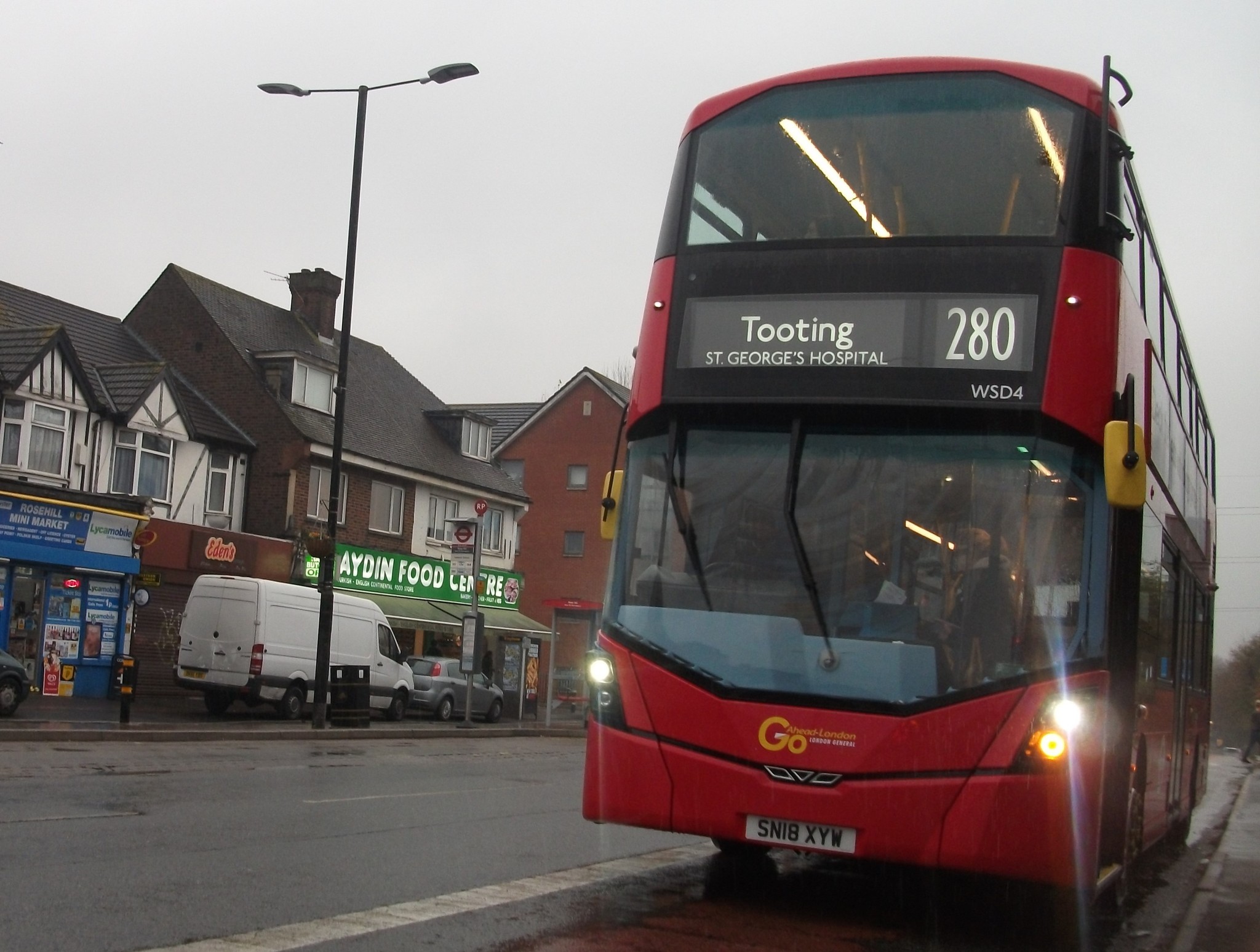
[{"x1": 1240, "y1": 757, "x2": 1252, "y2": 764}]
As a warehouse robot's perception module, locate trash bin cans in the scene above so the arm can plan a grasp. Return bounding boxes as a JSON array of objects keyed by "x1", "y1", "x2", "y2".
[{"x1": 330, "y1": 664, "x2": 370, "y2": 728}]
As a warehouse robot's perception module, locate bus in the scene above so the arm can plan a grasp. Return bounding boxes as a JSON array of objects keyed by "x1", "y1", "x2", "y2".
[{"x1": 581, "y1": 54, "x2": 1222, "y2": 930}]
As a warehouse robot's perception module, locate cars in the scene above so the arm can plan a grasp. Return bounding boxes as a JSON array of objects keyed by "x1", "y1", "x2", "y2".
[
  {"x1": 0, "y1": 647, "x2": 31, "y2": 717},
  {"x1": 405, "y1": 654, "x2": 504, "y2": 721}
]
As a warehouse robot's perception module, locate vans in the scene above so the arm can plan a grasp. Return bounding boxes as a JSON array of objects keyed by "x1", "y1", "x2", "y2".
[{"x1": 171, "y1": 573, "x2": 415, "y2": 722}]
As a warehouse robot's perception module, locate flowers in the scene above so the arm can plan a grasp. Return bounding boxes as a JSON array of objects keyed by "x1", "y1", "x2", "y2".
[{"x1": 302, "y1": 529, "x2": 330, "y2": 539}]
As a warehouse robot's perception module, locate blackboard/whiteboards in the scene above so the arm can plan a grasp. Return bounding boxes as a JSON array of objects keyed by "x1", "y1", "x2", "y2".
[{"x1": 107, "y1": 654, "x2": 138, "y2": 703}]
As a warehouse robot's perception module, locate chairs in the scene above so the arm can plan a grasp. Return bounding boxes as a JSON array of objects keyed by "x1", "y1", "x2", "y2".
[{"x1": 707, "y1": 519, "x2": 784, "y2": 580}]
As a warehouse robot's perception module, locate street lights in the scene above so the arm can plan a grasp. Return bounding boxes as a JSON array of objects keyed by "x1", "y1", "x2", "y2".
[{"x1": 253, "y1": 63, "x2": 482, "y2": 736}]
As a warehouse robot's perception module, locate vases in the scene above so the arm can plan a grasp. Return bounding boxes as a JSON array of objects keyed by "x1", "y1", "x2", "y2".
[{"x1": 305, "y1": 539, "x2": 334, "y2": 556}]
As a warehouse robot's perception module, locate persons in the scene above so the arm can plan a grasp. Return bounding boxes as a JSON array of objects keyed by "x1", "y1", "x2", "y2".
[{"x1": 1241, "y1": 700, "x2": 1260, "y2": 764}]
